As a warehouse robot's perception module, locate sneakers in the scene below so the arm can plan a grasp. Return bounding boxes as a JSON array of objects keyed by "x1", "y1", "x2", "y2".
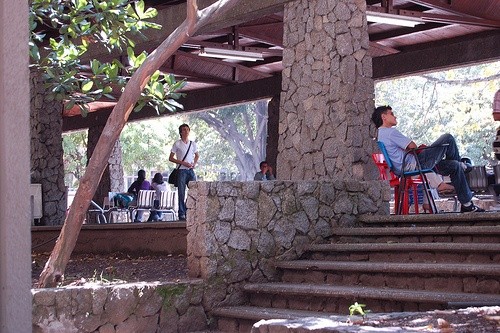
[
  {"x1": 461, "y1": 162, "x2": 472, "y2": 173},
  {"x1": 460, "y1": 200, "x2": 485, "y2": 213}
]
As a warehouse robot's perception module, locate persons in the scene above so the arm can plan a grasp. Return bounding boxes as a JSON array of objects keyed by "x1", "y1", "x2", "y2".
[
  {"x1": 372, "y1": 106, "x2": 485, "y2": 213},
  {"x1": 493, "y1": 90, "x2": 500, "y2": 121},
  {"x1": 152, "y1": 173, "x2": 170, "y2": 222},
  {"x1": 128, "y1": 170, "x2": 151, "y2": 223},
  {"x1": 254, "y1": 161, "x2": 275, "y2": 181},
  {"x1": 422, "y1": 171, "x2": 454, "y2": 213},
  {"x1": 169, "y1": 124, "x2": 199, "y2": 220}
]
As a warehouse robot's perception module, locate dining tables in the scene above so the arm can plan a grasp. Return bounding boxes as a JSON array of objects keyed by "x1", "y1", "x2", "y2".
[{"x1": 113, "y1": 193, "x2": 135, "y2": 223}]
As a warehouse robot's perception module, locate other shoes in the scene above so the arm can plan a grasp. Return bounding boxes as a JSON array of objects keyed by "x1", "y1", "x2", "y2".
[
  {"x1": 179, "y1": 215, "x2": 185, "y2": 219},
  {"x1": 153, "y1": 214, "x2": 163, "y2": 221}
]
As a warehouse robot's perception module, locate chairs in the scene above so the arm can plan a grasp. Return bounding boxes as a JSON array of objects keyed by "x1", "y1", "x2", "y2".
[
  {"x1": 88, "y1": 190, "x2": 176, "y2": 225},
  {"x1": 372, "y1": 140, "x2": 459, "y2": 214}
]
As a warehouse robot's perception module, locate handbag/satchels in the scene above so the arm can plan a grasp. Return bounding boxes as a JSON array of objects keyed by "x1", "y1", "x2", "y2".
[
  {"x1": 168, "y1": 168, "x2": 180, "y2": 187},
  {"x1": 111, "y1": 193, "x2": 134, "y2": 207}
]
